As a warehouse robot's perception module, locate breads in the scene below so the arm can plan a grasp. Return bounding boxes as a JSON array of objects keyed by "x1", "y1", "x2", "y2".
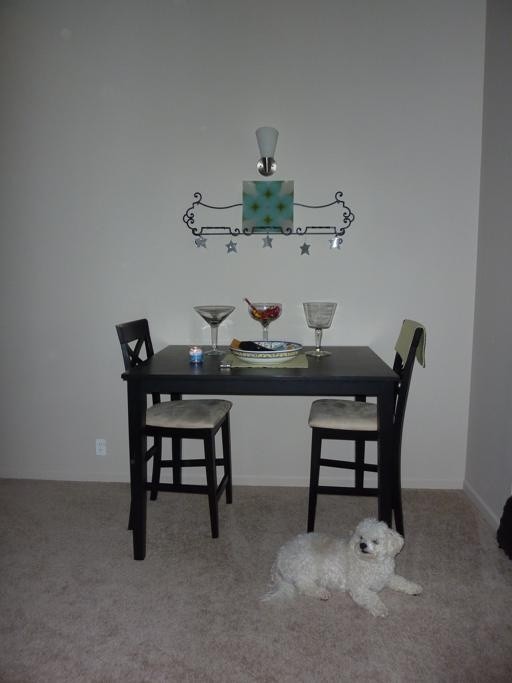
[{"x1": 230, "y1": 337, "x2": 240, "y2": 348}]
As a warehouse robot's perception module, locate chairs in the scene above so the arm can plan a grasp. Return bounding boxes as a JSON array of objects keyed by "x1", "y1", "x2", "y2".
[
  {"x1": 306, "y1": 316, "x2": 423, "y2": 540},
  {"x1": 116, "y1": 318, "x2": 233, "y2": 540}
]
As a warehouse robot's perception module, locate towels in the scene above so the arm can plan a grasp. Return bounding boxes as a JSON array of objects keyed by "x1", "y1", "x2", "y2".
[{"x1": 397, "y1": 316, "x2": 430, "y2": 370}]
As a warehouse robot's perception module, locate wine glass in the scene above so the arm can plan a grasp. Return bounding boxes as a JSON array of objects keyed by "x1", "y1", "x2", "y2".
[
  {"x1": 302, "y1": 300, "x2": 336, "y2": 358},
  {"x1": 193, "y1": 304, "x2": 236, "y2": 358},
  {"x1": 242, "y1": 296, "x2": 282, "y2": 350}
]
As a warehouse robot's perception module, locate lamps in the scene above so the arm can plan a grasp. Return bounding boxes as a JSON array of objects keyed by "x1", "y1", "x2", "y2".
[{"x1": 251, "y1": 125, "x2": 280, "y2": 173}]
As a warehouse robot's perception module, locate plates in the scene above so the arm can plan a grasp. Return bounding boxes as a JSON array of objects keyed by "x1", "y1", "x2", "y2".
[{"x1": 228, "y1": 340, "x2": 304, "y2": 364}]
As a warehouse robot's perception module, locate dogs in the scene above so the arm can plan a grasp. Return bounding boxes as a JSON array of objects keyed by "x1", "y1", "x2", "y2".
[{"x1": 259, "y1": 516, "x2": 423, "y2": 620}]
